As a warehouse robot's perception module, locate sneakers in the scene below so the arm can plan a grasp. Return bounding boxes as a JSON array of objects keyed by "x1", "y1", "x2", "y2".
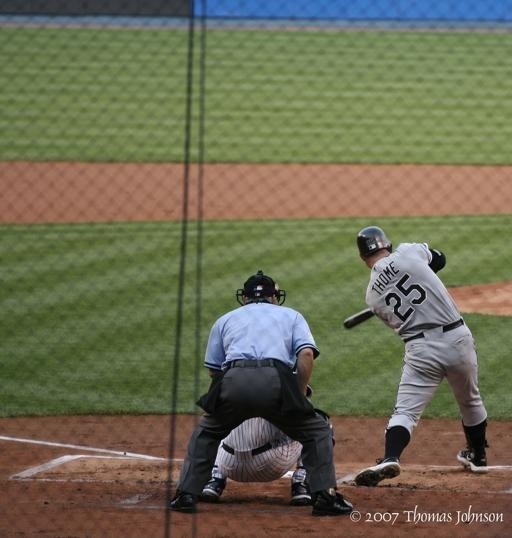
[
  {"x1": 290, "y1": 482, "x2": 312, "y2": 506},
  {"x1": 201, "y1": 478, "x2": 223, "y2": 502},
  {"x1": 312, "y1": 491, "x2": 353, "y2": 516},
  {"x1": 168, "y1": 492, "x2": 195, "y2": 513},
  {"x1": 457, "y1": 449, "x2": 488, "y2": 473},
  {"x1": 354, "y1": 456, "x2": 401, "y2": 487}
]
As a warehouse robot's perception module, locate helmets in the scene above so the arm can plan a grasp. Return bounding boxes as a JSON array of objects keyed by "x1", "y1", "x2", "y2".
[
  {"x1": 356, "y1": 226, "x2": 392, "y2": 257},
  {"x1": 235, "y1": 270, "x2": 286, "y2": 305}
]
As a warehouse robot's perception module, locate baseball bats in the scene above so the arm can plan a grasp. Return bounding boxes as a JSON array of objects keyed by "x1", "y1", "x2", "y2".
[{"x1": 343, "y1": 309, "x2": 373, "y2": 328}]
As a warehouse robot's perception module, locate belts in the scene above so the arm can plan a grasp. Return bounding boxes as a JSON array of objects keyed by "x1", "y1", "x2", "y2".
[
  {"x1": 222, "y1": 442, "x2": 272, "y2": 456},
  {"x1": 403, "y1": 319, "x2": 465, "y2": 344},
  {"x1": 231, "y1": 358, "x2": 274, "y2": 369}
]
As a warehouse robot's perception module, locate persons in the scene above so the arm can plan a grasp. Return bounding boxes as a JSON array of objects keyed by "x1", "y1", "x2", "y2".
[
  {"x1": 355, "y1": 225, "x2": 490, "y2": 486},
  {"x1": 169, "y1": 268, "x2": 353, "y2": 517},
  {"x1": 201, "y1": 416, "x2": 312, "y2": 506}
]
